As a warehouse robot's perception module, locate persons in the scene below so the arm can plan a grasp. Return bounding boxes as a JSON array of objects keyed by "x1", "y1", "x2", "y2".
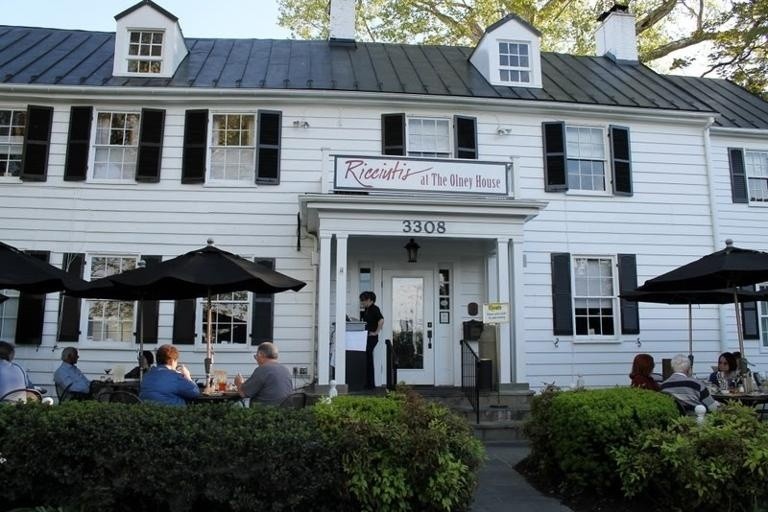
[
  {"x1": 54, "y1": 346, "x2": 94, "y2": 401},
  {"x1": 1, "y1": 340, "x2": 36, "y2": 403},
  {"x1": 708, "y1": 352, "x2": 744, "y2": 388},
  {"x1": 358, "y1": 291, "x2": 384, "y2": 390},
  {"x1": 125, "y1": 349, "x2": 155, "y2": 380},
  {"x1": 628, "y1": 353, "x2": 659, "y2": 392},
  {"x1": 234, "y1": 342, "x2": 294, "y2": 410},
  {"x1": 660, "y1": 353, "x2": 724, "y2": 415},
  {"x1": 137, "y1": 345, "x2": 200, "y2": 408}
]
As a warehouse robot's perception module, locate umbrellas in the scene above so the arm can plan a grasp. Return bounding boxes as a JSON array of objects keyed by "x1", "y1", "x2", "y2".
[
  {"x1": 108, "y1": 237, "x2": 308, "y2": 387},
  {"x1": 635, "y1": 238, "x2": 768, "y2": 359},
  {"x1": 0, "y1": 240, "x2": 91, "y2": 294},
  {"x1": 617, "y1": 283, "x2": 767, "y2": 359},
  {"x1": 61, "y1": 261, "x2": 199, "y2": 384}
]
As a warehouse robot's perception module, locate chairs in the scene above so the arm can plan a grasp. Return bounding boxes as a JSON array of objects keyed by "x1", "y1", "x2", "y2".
[
  {"x1": 98, "y1": 391, "x2": 113, "y2": 402},
  {"x1": 54, "y1": 382, "x2": 73, "y2": 404},
  {"x1": 109, "y1": 391, "x2": 144, "y2": 404},
  {"x1": 753, "y1": 401, "x2": 768, "y2": 413},
  {"x1": 661, "y1": 391, "x2": 686, "y2": 416},
  {"x1": 278, "y1": 392, "x2": 307, "y2": 411},
  {"x1": 1, "y1": 388, "x2": 42, "y2": 404},
  {"x1": 89, "y1": 380, "x2": 105, "y2": 400}
]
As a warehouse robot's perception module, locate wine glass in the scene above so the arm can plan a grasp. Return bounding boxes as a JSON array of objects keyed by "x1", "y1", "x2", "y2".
[{"x1": 104, "y1": 368, "x2": 112, "y2": 378}]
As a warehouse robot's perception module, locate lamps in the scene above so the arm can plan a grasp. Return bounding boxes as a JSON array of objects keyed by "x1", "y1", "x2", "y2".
[{"x1": 403, "y1": 238, "x2": 421, "y2": 262}]
